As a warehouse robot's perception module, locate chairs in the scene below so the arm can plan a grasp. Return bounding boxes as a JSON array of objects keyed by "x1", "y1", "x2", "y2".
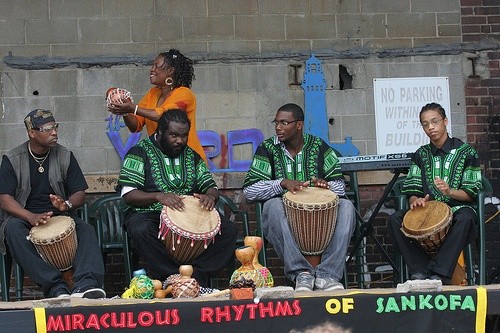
[
  {"x1": 0, "y1": 149, "x2": 365, "y2": 302},
  {"x1": 392, "y1": 172, "x2": 493, "y2": 286}
]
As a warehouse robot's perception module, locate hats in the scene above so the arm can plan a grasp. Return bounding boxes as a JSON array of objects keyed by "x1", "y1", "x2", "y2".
[{"x1": 24, "y1": 109, "x2": 55, "y2": 130}]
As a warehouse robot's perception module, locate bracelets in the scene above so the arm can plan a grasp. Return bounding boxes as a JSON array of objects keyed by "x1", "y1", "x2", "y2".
[
  {"x1": 134, "y1": 105, "x2": 138, "y2": 116},
  {"x1": 65, "y1": 201, "x2": 72, "y2": 209}
]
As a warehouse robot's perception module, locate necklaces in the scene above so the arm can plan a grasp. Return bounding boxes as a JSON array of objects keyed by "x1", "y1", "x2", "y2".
[{"x1": 29, "y1": 142, "x2": 49, "y2": 173}]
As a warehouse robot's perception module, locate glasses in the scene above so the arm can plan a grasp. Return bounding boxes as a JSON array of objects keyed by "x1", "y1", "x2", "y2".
[
  {"x1": 33, "y1": 123, "x2": 59, "y2": 132},
  {"x1": 271, "y1": 120, "x2": 297, "y2": 126},
  {"x1": 421, "y1": 117, "x2": 445, "y2": 127}
]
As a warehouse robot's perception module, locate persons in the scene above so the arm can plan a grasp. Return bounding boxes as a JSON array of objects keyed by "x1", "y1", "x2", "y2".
[
  {"x1": 0, "y1": 108, "x2": 106, "y2": 299},
  {"x1": 109, "y1": 48, "x2": 208, "y2": 168},
  {"x1": 118, "y1": 109, "x2": 238, "y2": 287},
  {"x1": 391, "y1": 103, "x2": 482, "y2": 281},
  {"x1": 242, "y1": 104, "x2": 356, "y2": 291}
]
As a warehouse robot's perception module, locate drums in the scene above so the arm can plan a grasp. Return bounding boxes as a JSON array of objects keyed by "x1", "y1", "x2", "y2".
[
  {"x1": 29, "y1": 215, "x2": 78, "y2": 289},
  {"x1": 159, "y1": 194, "x2": 221, "y2": 268},
  {"x1": 282, "y1": 186, "x2": 339, "y2": 270},
  {"x1": 402, "y1": 200, "x2": 466, "y2": 285}
]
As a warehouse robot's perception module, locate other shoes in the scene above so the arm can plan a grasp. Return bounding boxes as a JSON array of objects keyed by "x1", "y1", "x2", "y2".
[
  {"x1": 411, "y1": 272, "x2": 429, "y2": 280},
  {"x1": 294, "y1": 271, "x2": 316, "y2": 293},
  {"x1": 71, "y1": 287, "x2": 107, "y2": 300},
  {"x1": 430, "y1": 273, "x2": 447, "y2": 286},
  {"x1": 316, "y1": 274, "x2": 344, "y2": 291},
  {"x1": 52, "y1": 288, "x2": 71, "y2": 299}
]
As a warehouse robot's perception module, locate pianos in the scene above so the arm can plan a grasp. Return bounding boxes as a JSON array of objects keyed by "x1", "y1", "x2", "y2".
[{"x1": 337, "y1": 151, "x2": 417, "y2": 281}]
